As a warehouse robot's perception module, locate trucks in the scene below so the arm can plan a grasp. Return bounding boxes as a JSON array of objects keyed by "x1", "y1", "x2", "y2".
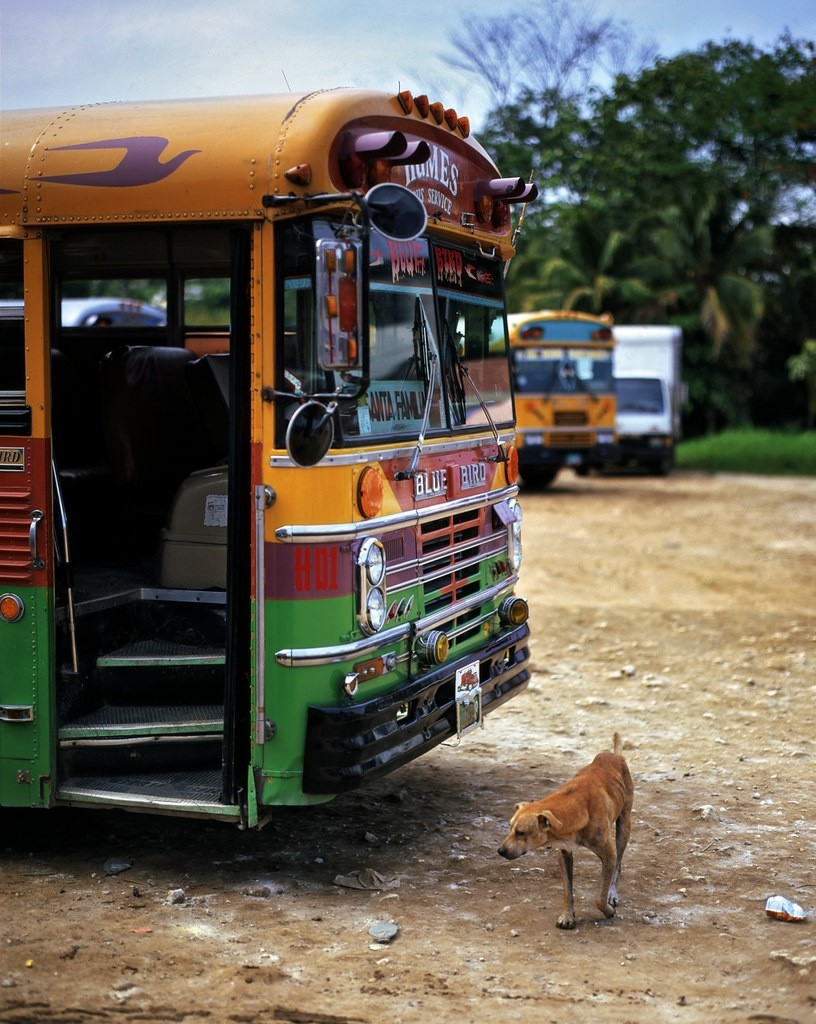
[{"x1": 570, "y1": 323, "x2": 683, "y2": 477}]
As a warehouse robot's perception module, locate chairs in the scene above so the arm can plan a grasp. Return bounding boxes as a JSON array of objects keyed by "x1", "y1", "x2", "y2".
[
  {"x1": 99, "y1": 343, "x2": 209, "y2": 508},
  {"x1": 50, "y1": 347, "x2": 114, "y2": 499}
]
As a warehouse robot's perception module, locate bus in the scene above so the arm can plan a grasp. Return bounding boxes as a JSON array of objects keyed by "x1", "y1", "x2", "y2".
[
  {"x1": 469, "y1": 307, "x2": 615, "y2": 492},
  {"x1": 0, "y1": 86, "x2": 535, "y2": 827}
]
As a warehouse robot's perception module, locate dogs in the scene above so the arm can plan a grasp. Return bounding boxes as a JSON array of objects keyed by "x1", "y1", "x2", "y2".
[{"x1": 497, "y1": 732, "x2": 634, "y2": 928}]
